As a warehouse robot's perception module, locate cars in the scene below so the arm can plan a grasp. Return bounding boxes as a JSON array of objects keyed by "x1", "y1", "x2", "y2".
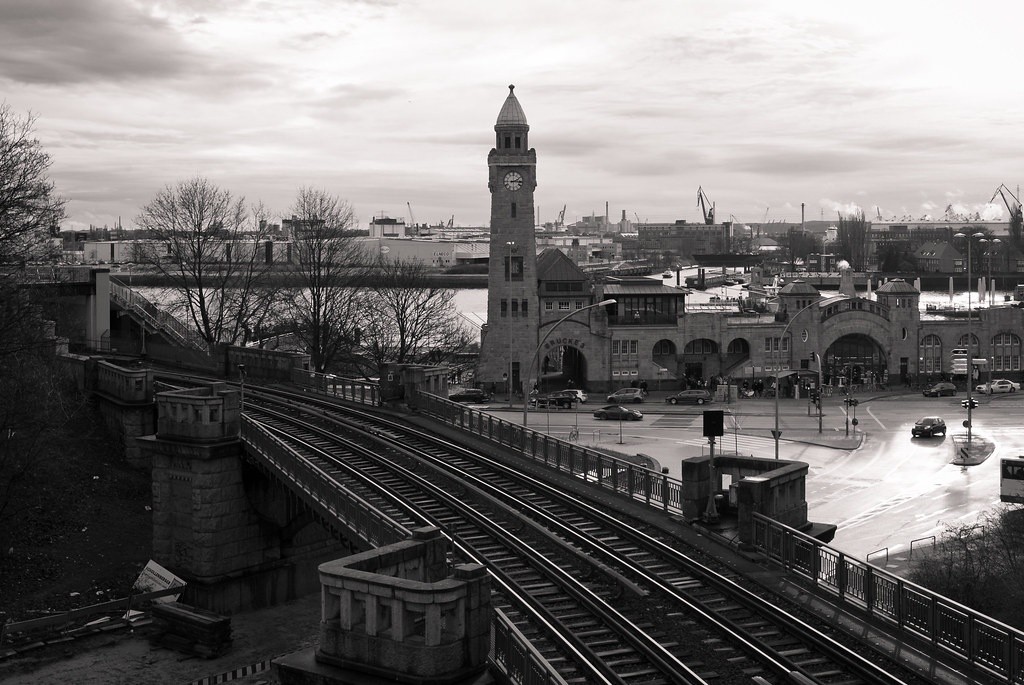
[
  {"x1": 922, "y1": 382, "x2": 958, "y2": 397},
  {"x1": 606, "y1": 388, "x2": 645, "y2": 403},
  {"x1": 593, "y1": 404, "x2": 643, "y2": 420},
  {"x1": 911, "y1": 415, "x2": 947, "y2": 439},
  {"x1": 529, "y1": 392, "x2": 583, "y2": 408},
  {"x1": 975, "y1": 379, "x2": 1021, "y2": 395},
  {"x1": 552, "y1": 389, "x2": 588, "y2": 402}
]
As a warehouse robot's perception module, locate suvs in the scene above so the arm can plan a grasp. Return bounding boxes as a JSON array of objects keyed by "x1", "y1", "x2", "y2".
[
  {"x1": 665, "y1": 389, "x2": 712, "y2": 405},
  {"x1": 449, "y1": 388, "x2": 484, "y2": 403}
]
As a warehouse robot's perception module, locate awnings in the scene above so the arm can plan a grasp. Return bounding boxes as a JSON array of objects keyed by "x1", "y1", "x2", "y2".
[{"x1": 770, "y1": 370, "x2": 797, "y2": 379}]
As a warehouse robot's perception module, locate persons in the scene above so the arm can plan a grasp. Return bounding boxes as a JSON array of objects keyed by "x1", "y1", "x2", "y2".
[
  {"x1": 634, "y1": 310, "x2": 641, "y2": 324},
  {"x1": 743, "y1": 379, "x2": 763, "y2": 396},
  {"x1": 772, "y1": 380, "x2": 777, "y2": 391},
  {"x1": 628, "y1": 377, "x2": 648, "y2": 396},
  {"x1": 451, "y1": 368, "x2": 463, "y2": 385},
  {"x1": 530, "y1": 382, "x2": 539, "y2": 396},
  {"x1": 685, "y1": 372, "x2": 723, "y2": 390}
]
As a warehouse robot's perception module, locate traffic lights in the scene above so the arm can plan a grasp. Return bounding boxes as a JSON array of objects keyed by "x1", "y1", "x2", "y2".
[
  {"x1": 849, "y1": 399, "x2": 854, "y2": 406},
  {"x1": 809, "y1": 351, "x2": 815, "y2": 362},
  {"x1": 961, "y1": 400, "x2": 969, "y2": 407},
  {"x1": 811, "y1": 392, "x2": 816, "y2": 404},
  {"x1": 972, "y1": 399, "x2": 979, "y2": 409}
]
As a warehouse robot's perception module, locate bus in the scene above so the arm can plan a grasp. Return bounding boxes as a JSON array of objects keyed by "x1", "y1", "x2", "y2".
[{"x1": 999, "y1": 455, "x2": 1024, "y2": 506}]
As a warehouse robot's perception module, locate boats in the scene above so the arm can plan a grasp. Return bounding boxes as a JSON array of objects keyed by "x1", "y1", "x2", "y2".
[{"x1": 661, "y1": 266, "x2": 674, "y2": 278}]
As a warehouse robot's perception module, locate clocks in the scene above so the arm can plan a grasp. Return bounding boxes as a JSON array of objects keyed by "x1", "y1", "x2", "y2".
[{"x1": 503, "y1": 169, "x2": 523, "y2": 192}]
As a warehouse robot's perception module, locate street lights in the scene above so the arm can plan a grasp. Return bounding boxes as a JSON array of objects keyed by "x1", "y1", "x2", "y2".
[
  {"x1": 979, "y1": 238, "x2": 1002, "y2": 380},
  {"x1": 523, "y1": 297, "x2": 617, "y2": 424},
  {"x1": 816, "y1": 354, "x2": 825, "y2": 433},
  {"x1": 953, "y1": 232, "x2": 985, "y2": 446},
  {"x1": 506, "y1": 240, "x2": 515, "y2": 408},
  {"x1": 768, "y1": 291, "x2": 851, "y2": 460}
]
